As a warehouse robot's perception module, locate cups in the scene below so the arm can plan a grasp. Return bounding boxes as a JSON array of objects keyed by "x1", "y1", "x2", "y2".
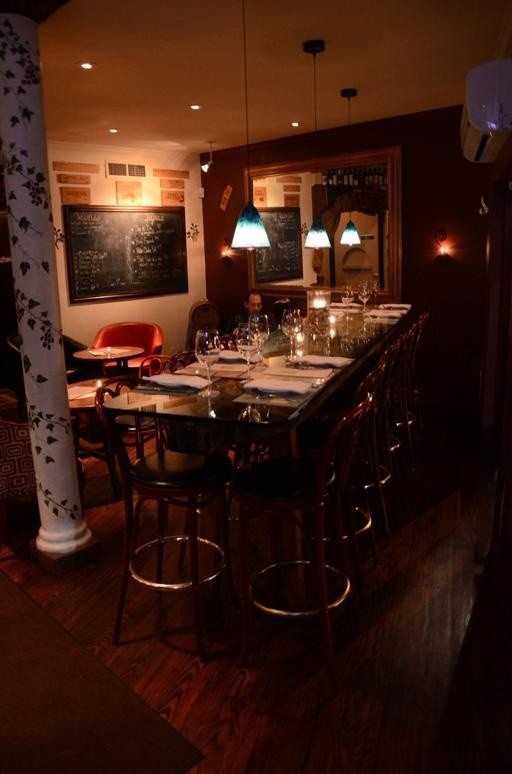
[{"x1": 304, "y1": 289, "x2": 332, "y2": 340}]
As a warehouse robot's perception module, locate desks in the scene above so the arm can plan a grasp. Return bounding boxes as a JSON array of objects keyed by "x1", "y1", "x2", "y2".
[
  {"x1": 67, "y1": 379, "x2": 131, "y2": 501},
  {"x1": 72, "y1": 343, "x2": 145, "y2": 377}
]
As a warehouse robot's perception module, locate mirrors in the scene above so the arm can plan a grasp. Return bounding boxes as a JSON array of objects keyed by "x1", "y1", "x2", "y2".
[{"x1": 237, "y1": 142, "x2": 403, "y2": 303}]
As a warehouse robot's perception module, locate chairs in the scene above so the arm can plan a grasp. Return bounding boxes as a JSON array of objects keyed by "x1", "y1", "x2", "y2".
[{"x1": 1, "y1": 331, "x2": 88, "y2": 503}]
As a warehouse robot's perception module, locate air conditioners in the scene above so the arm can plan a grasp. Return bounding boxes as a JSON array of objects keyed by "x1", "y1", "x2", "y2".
[{"x1": 459, "y1": 59, "x2": 512, "y2": 164}]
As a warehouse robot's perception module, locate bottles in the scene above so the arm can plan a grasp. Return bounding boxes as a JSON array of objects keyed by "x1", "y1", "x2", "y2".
[{"x1": 321, "y1": 165, "x2": 387, "y2": 187}]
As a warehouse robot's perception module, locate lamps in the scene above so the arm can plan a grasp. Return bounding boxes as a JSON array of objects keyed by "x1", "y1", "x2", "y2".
[
  {"x1": 201, "y1": 141, "x2": 214, "y2": 175},
  {"x1": 229, "y1": 1, "x2": 271, "y2": 251},
  {"x1": 303, "y1": 39, "x2": 361, "y2": 250}
]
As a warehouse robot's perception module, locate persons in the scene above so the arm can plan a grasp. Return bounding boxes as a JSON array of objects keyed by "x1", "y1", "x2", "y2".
[{"x1": 228, "y1": 290, "x2": 274, "y2": 336}]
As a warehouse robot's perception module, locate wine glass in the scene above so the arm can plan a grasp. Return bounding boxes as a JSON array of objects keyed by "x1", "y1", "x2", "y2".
[
  {"x1": 340, "y1": 285, "x2": 354, "y2": 321},
  {"x1": 357, "y1": 279, "x2": 382, "y2": 314},
  {"x1": 234, "y1": 322, "x2": 262, "y2": 386},
  {"x1": 247, "y1": 312, "x2": 271, "y2": 368},
  {"x1": 194, "y1": 327, "x2": 222, "y2": 399},
  {"x1": 281, "y1": 307, "x2": 302, "y2": 363}
]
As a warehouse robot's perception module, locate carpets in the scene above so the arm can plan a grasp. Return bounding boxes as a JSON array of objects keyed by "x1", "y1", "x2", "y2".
[{"x1": 0, "y1": 569, "x2": 206, "y2": 773}]
